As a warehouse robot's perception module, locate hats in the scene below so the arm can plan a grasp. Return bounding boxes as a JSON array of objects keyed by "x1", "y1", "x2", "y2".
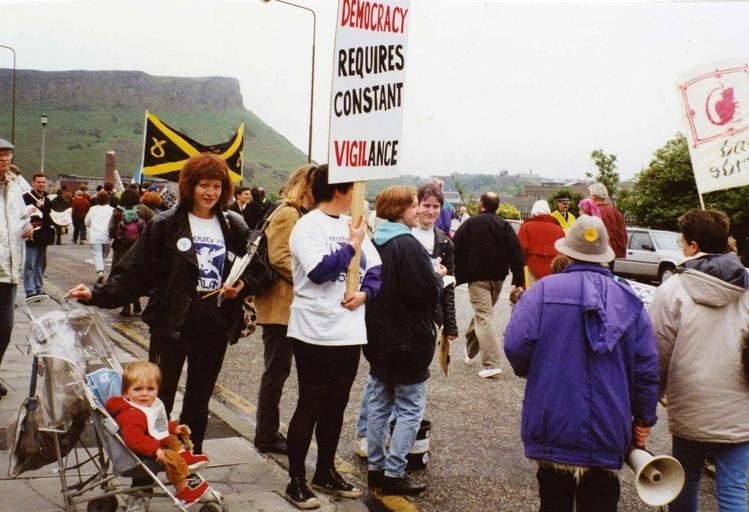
[
  {"x1": 0, "y1": 138, "x2": 16, "y2": 151},
  {"x1": 555, "y1": 194, "x2": 570, "y2": 204},
  {"x1": 552, "y1": 214, "x2": 617, "y2": 268}
]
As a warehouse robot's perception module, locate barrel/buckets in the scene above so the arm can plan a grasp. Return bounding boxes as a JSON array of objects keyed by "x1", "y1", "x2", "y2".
[{"x1": 391, "y1": 420, "x2": 431, "y2": 470}]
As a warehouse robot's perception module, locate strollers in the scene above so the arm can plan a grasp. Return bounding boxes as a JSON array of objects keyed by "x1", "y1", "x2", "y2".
[{"x1": 20, "y1": 290, "x2": 229, "y2": 512}]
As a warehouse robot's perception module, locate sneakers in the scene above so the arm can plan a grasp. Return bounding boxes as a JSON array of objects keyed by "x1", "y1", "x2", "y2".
[
  {"x1": 464, "y1": 344, "x2": 474, "y2": 366},
  {"x1": 119, "y1": 302, "x2": 142, "y2": 317},
  {"x1": 310, "y1": 462, "x2": 362, "y2": 500},
  {"x1": 50, "y1": 230, "x2": 85, "y2": 245},
  {"x1": 477, "y1": 367, "x2": 502, "y2": 378},
  {"x1": 283, "y1": 474, "x2": 320, "y2": 509},
  {"x1": 353, "y1": 436, "x2": 369, "y2": 459},
  {"x1": 177, "y1": 449, "x2": 209, "y2": 471},
  {"x1": 367, "y1": 471, "x2": 427, "y2": 496},
  {"x1": 95, "y1": 272, "x2": 104, "y2": 284},
  {"x1": 252, "y1": 433, "x2": 289, "y2": 456},
  {"x1": 173, "y1": 482, "x2": 210, "y2": 511}
]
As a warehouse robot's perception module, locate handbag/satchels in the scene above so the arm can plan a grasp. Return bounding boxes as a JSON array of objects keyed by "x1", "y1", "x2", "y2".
[
  {"x1": 236, "y1": 294, "x2": 259, "y2": 337},
  {"x1": 6, "y1": 397, "x2": 90, "y2": 482}
]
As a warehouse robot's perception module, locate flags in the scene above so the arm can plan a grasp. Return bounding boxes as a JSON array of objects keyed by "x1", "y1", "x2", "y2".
[
  {"x1": 139, "y1": 107, "x2": 246, "y2": 190},
  {"x1": 674, "y1": 60, "x2": 749, "y2": 195}
]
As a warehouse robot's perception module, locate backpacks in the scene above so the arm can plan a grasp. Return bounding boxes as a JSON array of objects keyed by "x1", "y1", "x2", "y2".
[
  {"x1": 115, "y1": 204, "x2": 145, "y2": 244},
  {"x1": 240, "y1": 199, "x2": 295, "y2": 297}
]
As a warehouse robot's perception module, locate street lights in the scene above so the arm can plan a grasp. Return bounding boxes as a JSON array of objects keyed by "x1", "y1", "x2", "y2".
[
  {"x1": 37, "y1": 111, "x2": 48, "y2": 177},
  {"x1": 259, "y1": 0, "x2": 316, "y2": 165}
]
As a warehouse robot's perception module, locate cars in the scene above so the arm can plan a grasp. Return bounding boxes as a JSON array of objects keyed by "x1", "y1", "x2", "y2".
[{"x1": 608, "y1": 228, "x2": 697, "y2": 286}]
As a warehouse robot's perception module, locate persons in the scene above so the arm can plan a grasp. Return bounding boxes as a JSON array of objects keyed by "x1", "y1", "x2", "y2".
[
  {"x1": 58, "y1": 151, "x2": 280, "y2": 511},
  {"x1": 641, "y1": 205, "x2": 748, "y2": 511},
  {"x1": 283, "y1": 162, "x2": 384, "y2": 511},
  {"x1": 351, "y1": 182, "x2": 459, "y2": 462},
  {"x1": 242, "y1": 162, "x2": 322, "y2": 455},
  {"x1": 0, "y1": 135, "x2": 34, "y2": 403},
  {"x1": 357, "y1": 182, "x2": 444, "y2": 495},
  {"x1": 501, "y1": 211, "x2": 661, "y2": 511},
  {"x1": 100, "y1": 358, "x2": 224, "y2": 505}
]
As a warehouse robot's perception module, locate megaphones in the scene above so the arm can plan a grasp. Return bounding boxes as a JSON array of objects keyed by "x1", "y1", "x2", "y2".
[{"x1": 624, "y1": 419, "x2": 685, "y2": 507}]
